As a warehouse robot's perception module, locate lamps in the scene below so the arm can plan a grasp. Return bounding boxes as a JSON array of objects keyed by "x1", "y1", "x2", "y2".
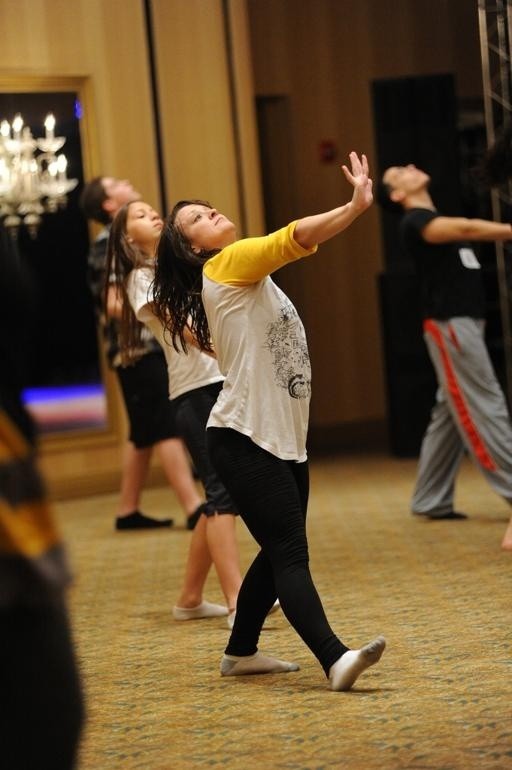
[{"x1": 1, "y1": 113, "x2": 81, "y2": 251}]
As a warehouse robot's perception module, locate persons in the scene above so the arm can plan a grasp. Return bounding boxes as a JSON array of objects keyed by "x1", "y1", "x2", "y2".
[
  {"x1": 83, "y1": 175, "x2": 204, "y2": 529},
  {"x1": 381, "y1": 163, "x2": 512, "y2": 519},
  {"x1": 0, "y1": 389, "x2": 85, "y2": 770},
  {"x1": 102, "y1": 200, "x2": 280, "y2": 630},
  {"x1": 153, "y1": 151, "x2": 387, "y2": 692}
]
